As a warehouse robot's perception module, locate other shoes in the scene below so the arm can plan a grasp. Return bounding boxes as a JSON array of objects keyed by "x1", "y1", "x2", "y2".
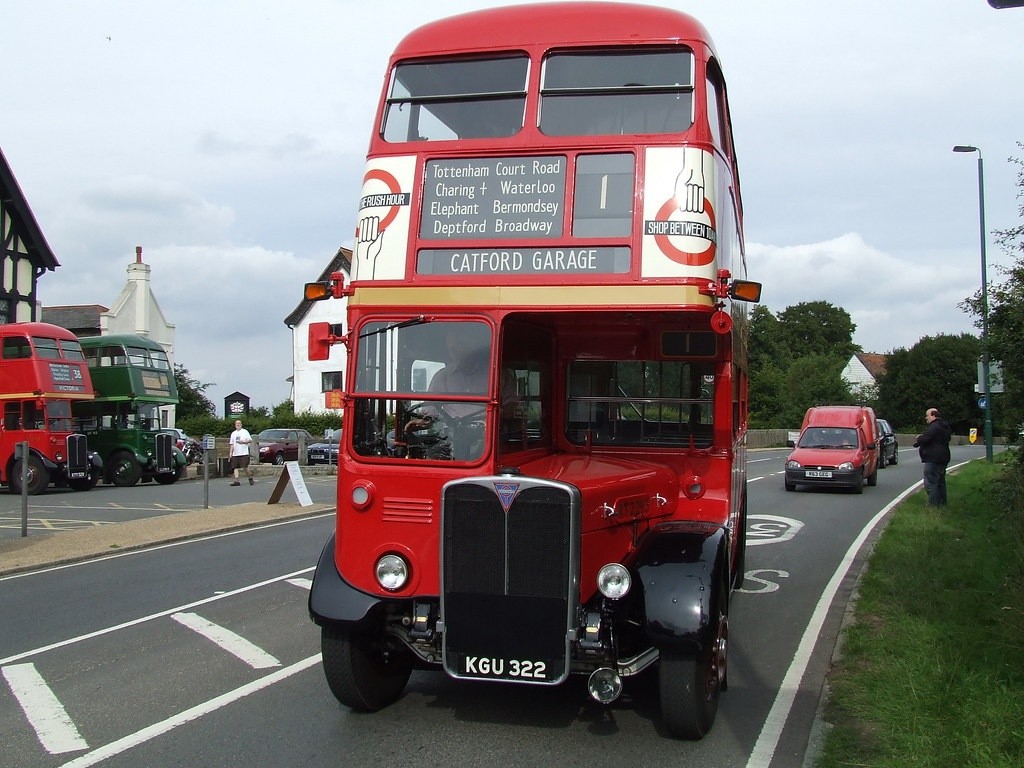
[
  {"x1": 230, "y1": 481, "x2": 240, "y2": 486},
  {"x1": 249, "y1": 478, "x2": 254, "y2": 485}
]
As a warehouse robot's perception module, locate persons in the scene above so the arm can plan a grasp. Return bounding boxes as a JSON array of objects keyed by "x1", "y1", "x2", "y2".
[
  {"x1": 809, "y1": 432, "x2": 824, "y2": 446},
  {"x1": 914, "y1": 408, "x2": 952, "y2": 510},
  {"x1": 229, "y1": 420, "x2": 254, "y2": 486},
  {"x1": 402, "y1": 325, "x2": 518, "y2": 455}
]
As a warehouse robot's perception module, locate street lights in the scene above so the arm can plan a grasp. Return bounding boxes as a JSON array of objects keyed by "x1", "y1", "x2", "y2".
[{"x1": 952, "y1": 144, "x2": 995, "y2": 463}]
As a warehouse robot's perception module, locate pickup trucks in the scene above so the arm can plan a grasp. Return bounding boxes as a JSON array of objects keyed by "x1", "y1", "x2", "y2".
[{"x1": 784, "y1": 405, "x2": 885, "y2": 494}]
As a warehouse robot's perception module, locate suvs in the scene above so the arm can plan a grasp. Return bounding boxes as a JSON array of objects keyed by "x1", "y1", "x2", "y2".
[{"x1": 151, "y1": 428, "x2": 205, "y2": 464}]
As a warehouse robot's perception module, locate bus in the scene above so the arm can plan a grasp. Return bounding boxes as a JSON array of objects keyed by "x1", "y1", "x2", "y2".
[
  {"x1": 301, "y1": 0, "x2": 762, "y2": 743},
  {"x1": 3, "y1": 334, "x2": 191, "y2": 487},
  {"x1": 0, "y1": 321, "x2": 103, "y2": 494}
]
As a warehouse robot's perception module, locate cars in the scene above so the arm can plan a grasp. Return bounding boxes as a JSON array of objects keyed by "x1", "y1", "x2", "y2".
[
  {"x1": 248, "y1": 429, "x2": 315, "y2": 465},
  {"x1": 876, "y1": 419, "x2": 900, "y2": 468},
  {"x1": 307, "y1": 428, "x2": 343, "y2": 466}
]
